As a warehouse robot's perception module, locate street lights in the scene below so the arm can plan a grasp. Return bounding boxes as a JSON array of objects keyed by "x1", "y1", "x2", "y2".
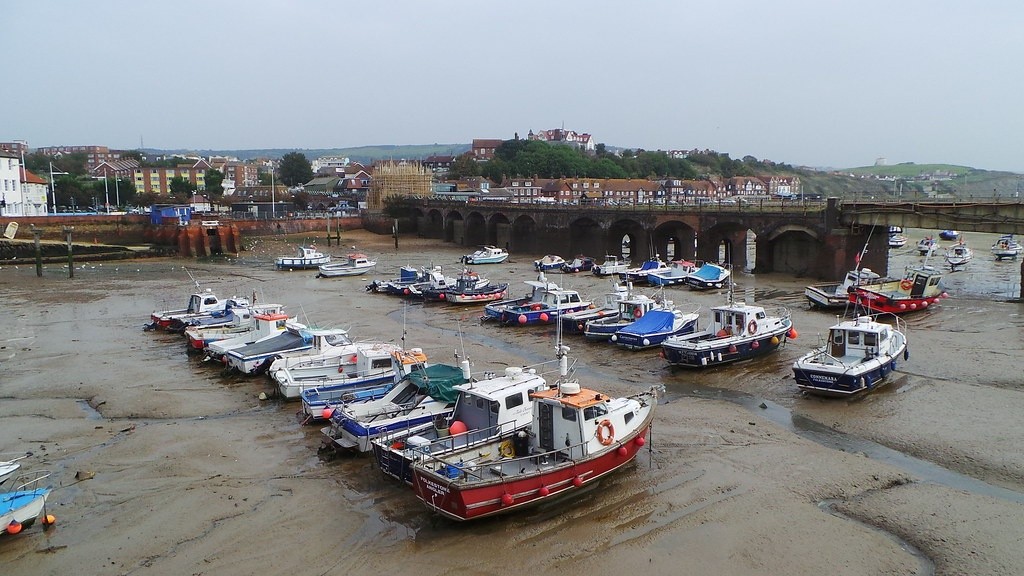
[
  {"x1": 91, "y1": 168, "x2": 110, "y2": 213},
  {"x1": 49, "y1": 161, "x2": 69, "y2": 213}
]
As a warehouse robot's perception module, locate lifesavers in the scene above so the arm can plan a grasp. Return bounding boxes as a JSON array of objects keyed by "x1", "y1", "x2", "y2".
[
  {"x1": 901, "y1": 280, "x2": 911, "y2": 290},
  {"x1": 500, "y1": 442, "x2": 515, "y2": 457},
  {"x1": 748, "y1": 319, "x2": 757, "y2": 334},
  {"x1": 634, "y1": 308, "x2": 642, "y2": 318},
  {"x1": 598, "y1": 420, "x2": 615, "y2": 445}
]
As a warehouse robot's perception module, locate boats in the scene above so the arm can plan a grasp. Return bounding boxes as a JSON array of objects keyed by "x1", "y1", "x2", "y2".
[
  {"x1": 371, "y1": 318, "x2": 570, "y2": 486},
  {"x1": 661, "y1": 241, "x2": 797, "y2": 368},
  {"x1": 0, "y1": 462, "x2": 21, "y2": 483},
  {"x1": 0, "y1": 470, "x2": 56, "y2": 536},
  {"x1": 590, "y1": 254, "x2": 632, "y2": 276},
  {"x1": 274, "y1": 237, "x2": 331, "y2": 270},
  {"x1": 992, "y1": 233, "x2": 1024, "y2": 262},
  {"x1": 301, "y1": 355, "x2": 431, "y2": 420},
  {"x1": 939, "y1": 230, "x2": 960, "y2": 241},
  {"x1": 560, "y1": 252, "x2": 596, "y2": 274},
  {"x1": 608, "y1": 284, "x2": 701, "y2": 352},
  {"x1": 791, "y1": 295, "x2": 909, "y2": 395},
  {"x1": 150, "y1": 265, "x2": 408, "y2": 399},
  {"x1": 805, "y1": 213, "x2": 902, "y2": 309},
  {"x1": 846, "y1": 244, "x2": 948, "y2": 314},
  {"x1": 319, "y1": 363, "x2": 505, "y2": 455},
  {"x1": 534, "y1": 255, "x2": 566, "y2": 271},
  {"x1": 916, "y1": 234, "x2": 941, "y2": 253},
  {"x1": 888, "y1": 235, "x2": 909, "y2": 247},
  {"x1": 582, "y1": 269, "x2": 665, "y2": 341},
  {"x1": 942, "y1": 235, "x2": 974, "y2": 266},
  {"x1": 366, "y1": 262, "x2": 666, "y2": 335},
  {"x1": 686, "y1": 259, "x2": 734, "y2": 290},
  {"x1": 619, "y1": 254, "x2": 701, "y2": 287},
  {"x1": 408, "y1": 273, "x2": 660, "y2": 521},
  {"x1": 318, "y1": 245, "x2": 378, "y2": 277},
  {"x1": 460, "y1": 245, "x2": 509, "y2": 265}
]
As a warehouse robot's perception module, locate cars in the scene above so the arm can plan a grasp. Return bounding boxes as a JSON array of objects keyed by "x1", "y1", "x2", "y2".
[{"x1": 537, "y1": 196, "x2": 750, "y2": 207}]
